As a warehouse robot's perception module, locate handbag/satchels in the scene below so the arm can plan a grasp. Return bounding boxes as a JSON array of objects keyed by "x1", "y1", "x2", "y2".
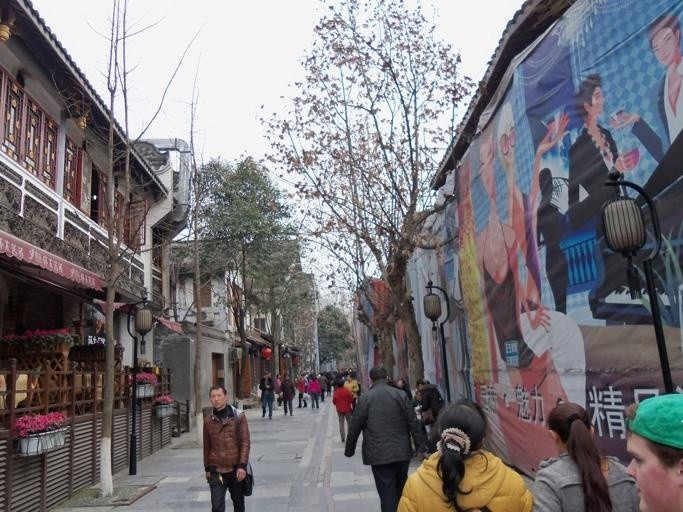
[
  {"x1": 420, "y1": 408, "x2": 435, "y2": 425},
  {"x1": 239, "y1": 461, "x2": 255, "y2": 497}
]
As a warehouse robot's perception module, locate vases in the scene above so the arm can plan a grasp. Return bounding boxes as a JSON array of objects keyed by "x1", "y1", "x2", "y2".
[
  {"x1": 155, "y1": 405, "x2": 173, "y2": 418},
  {"x1": 136, "y1": 384, "x2": 154, "y2": 399},
  {"x1": 15, "y1": 428, "x2": 67, "y2": 453}
]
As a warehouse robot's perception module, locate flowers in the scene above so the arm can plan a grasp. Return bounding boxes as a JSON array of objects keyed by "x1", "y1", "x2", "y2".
[
  {"x1": 128, "y1": 373, "x2": 157, "y2": 387},
  {"x1": 11, "y1": 412, "x2": 67, "y2": 439},
  {"x1": 154, "y1": 395, "x2": 174, "y2": 405}
]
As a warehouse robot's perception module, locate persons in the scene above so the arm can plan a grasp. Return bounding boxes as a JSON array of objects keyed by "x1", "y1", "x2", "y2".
[
  {"x1": 531, "y1": 401, "x2": 641, "y2": 512},
  {"x1": 259, "y1": 368, "x2": 445, "y2": 512},
  {"x1": 396, "y1": 398, "x2": 533, "y2": 512},
  {"x1": 203, "y1": 385, "x2": 250, "y2": 512},
  {"x1": 626, "y1": 393, "x2": 683, "y2": 512}
]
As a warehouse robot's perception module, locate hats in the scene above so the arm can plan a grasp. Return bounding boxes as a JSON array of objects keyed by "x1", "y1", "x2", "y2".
[{"x1": 624, "y1": 392, "x2": 683, "y2": 455}]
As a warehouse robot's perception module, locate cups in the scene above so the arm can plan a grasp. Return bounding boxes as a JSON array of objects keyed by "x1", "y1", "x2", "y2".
[{"x1": 618, "y1": 142, "x2": 640, "y2": 169}]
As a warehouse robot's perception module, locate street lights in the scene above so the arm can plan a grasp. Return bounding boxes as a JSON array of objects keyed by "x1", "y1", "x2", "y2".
[
  {"x1": 126, "y1": 285, "x2": 162, "y2": 477},
  {"x1": 421, "y1": 275, "x2": 454, "y2": 403},
  {"x1": 279, "y1": 345, "x2": 291, "y2": 374},
  {"x1": 589, "y1": 160, "x2": 678, "y2": 395}
]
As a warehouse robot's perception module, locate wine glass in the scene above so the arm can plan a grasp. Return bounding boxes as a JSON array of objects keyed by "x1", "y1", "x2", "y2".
[
  {"x1": 609, "y1": 106, "x2": 631, "y2": 146},
  {"x1": 540, "y1": 104, "x2": 568, "y2": 167}
]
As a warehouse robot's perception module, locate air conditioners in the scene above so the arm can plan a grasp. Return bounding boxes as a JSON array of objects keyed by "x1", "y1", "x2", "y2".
[{"x1": 200, "y1": 310, "x2": 214, "y2": 322}]
